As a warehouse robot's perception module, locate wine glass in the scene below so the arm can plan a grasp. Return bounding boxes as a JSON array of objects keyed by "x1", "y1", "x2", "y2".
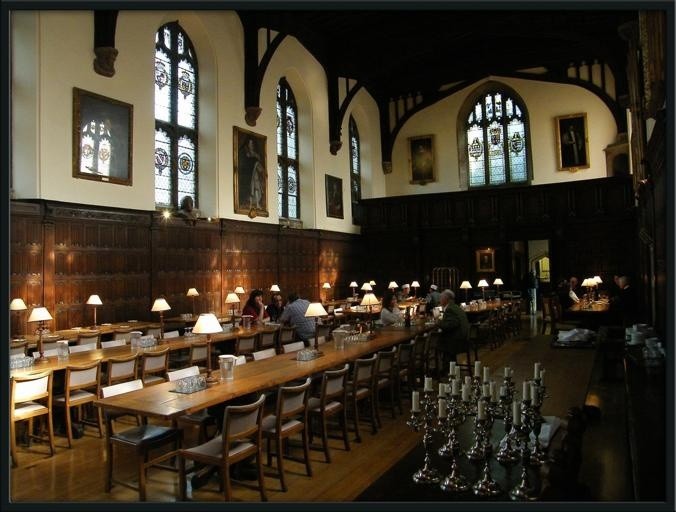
[{"x1": 184, "y1": 327, "x2": 199, "y2": 338}]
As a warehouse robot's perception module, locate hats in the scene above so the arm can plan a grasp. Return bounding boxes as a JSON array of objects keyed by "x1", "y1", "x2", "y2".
[
  {"x1": 431, "y1": 285, "x2": 438, "y2": 290},
  {"x1": 402, "y1": 284, "x2": 409, "y2": 289}
]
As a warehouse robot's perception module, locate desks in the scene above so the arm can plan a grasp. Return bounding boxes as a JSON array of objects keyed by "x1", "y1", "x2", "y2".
[{"x1": 349, "y1": 335, "x2": 595, "y2": 501}]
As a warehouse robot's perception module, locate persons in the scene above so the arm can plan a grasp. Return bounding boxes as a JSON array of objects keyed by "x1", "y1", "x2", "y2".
[
  {"x1": 568, "y1": 276, "x2": 587, "y2": 296},
  {"x1": 614, "y1": 276, "x2": 638, "y2": 318},
  {"x1": 426, "y1": 284, "x2": 440, "y2": 310},
  {"x1": 413, "y1": 144, "x2": 432, "y2": 176},
  {"x1": 398, "y1": 284, "x2": 414, "y2": 302},
  {"x1": 557, "y1": 279, "x2": 580, "y2": 307},
  {"x1": 267, "y1": 293, "x2": 284, "y2": 320},
  {"x1": 237, "y1": 134, "x2": 266, "y2": 212},
  {"x1": 280, "y1": 295, "x2": 316, "y2": 338},
  {"x1": 562, "y1": 124, "x2": 583, "y2": 165},
  {"x1": 614, "y1": 271, "x2": 625, "y2": 292},
  {"x1": 434, "y1": 289, "x2": 468, "y2": 347},
  {"x1": 381, "y1": 293, "x2": 400, "y2": 326},
  {"x1": 242, "y1": 289, "x2": 270, "y2": 324},
  {"x1": 330, "y1": 182, "x2": 340, "y2": 213}
]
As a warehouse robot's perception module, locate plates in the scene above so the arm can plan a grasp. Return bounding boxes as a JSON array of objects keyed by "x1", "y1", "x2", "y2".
[
  {"x1": 12, "y1": 339, "x2": 26, "y2": 343},
  {"x1": 47, "y1": 335, "x2": 59, "y2": 338},
  {"x1": 71, "y1": 327, "x2": 82, "y2": 330},
  {"x1": 120, "y1": 325, "x2": 130, "y2": 328},
  {"x1": 101, "y1": 324, "x2": 112, "y2": 326},
  {"x1": 128, "y1": 320, "x2": 138, "y2": 322},
  {"x1": 88, "y1": 330, "x2": 99, "y2": 332}
]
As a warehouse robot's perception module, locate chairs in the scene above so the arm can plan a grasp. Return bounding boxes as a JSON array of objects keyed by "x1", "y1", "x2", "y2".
[
  {"x1": 10, "y1": 292, "x2": 523, "y2": 503},
  {"x1": 540, "y1": 294, "x2": 567, "y2": 336}
]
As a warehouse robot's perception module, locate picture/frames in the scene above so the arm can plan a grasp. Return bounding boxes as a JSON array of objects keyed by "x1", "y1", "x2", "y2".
[
  {"x1": 553, "y1": 110, "x2": 590, "y2": 173},
  {"x1": 71, "y1": 87, "x2": 134, "y2": 187},
  {"x1": 406, "y1": 133, "x2": 439, "y2": 185},
  {"x1": 231, "y1": 125, "x2": 268, "y2": 216},
  {"x1": 322, "y1": 173, "x2": 343, "y2": 218},
  {"x1": 475, "y1": 248, "x2": 495, "y2": 273}
]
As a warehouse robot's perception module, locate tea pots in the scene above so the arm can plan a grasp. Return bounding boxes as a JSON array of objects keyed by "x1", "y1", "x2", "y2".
[
  {"x1": 217, "y1": 355, "x2": 237, "y2": 380},
  {"x1": 332, "y1": 328, "x2": 345, "y2": 349},
  {"x1": 128, "y1": 331, "x2": 142, "y2": 351},
  {"x1": 56, "y1": 340, "x2": 71, "y2": 361},
  {"x1": 242, "y1": 314, "x2": 254, "y2": 328}
]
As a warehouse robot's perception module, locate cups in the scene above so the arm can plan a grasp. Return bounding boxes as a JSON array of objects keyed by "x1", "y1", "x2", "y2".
[
  {"x1": 346, "y1": 334, "x2": 363, "y2": 345},
  {"x1": 174, "y1": 375, "x2": 207, "y2": 394},
  {"x1": 140, "y1": 335, "x2": 158, "y2": 348},
  {"x1": 10, "y1": 356, "x2": 33, "y2": 369},
  {"x1": 297, "y1": 347, "x2": 318, "y2": 360}
]
